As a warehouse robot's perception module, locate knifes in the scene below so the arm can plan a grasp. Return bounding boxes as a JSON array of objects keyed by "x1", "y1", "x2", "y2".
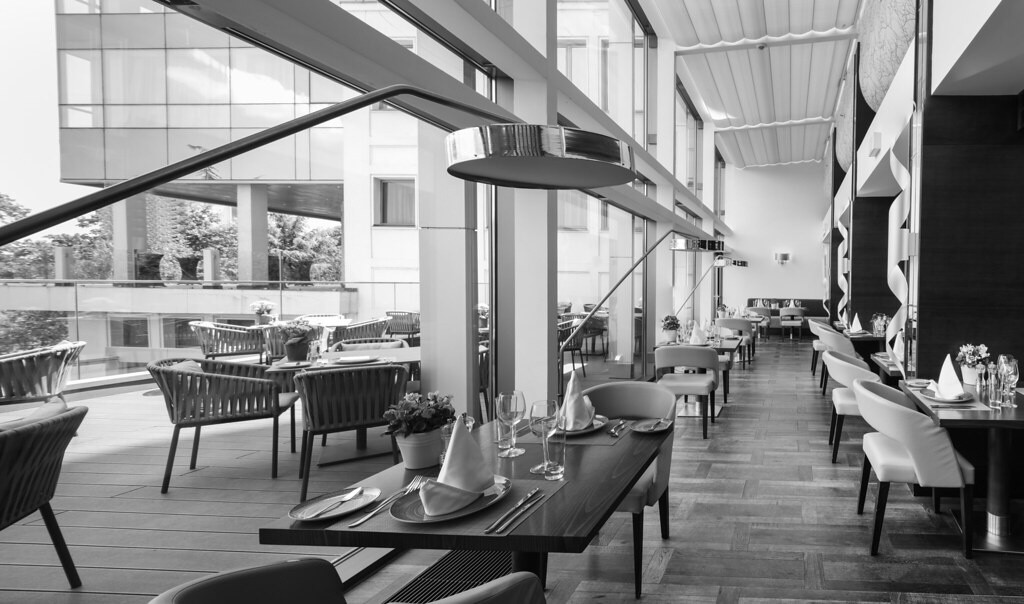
[
  {"x1": 495, "y1": 494, "x2": 544, "y2": 533},
  {"x1": 483, "y1": 487, "x2": 539, "y2": 533},
  {"x1": 931, "y1": 404, "x2": 976, "y2": 408},
  {"x1": 649, "y1": 421, "x2": 660, "y2": 430}
]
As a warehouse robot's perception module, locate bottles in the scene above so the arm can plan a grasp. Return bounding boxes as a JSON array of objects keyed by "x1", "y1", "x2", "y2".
[{"x1": 976, "y1": 362, "x2": 996, "y2": 385}]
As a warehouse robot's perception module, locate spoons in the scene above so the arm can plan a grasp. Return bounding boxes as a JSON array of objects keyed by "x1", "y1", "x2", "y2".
[
  {"x1": 306, "y1": 486, "x2": 361, "y2": 518},
  {"x1": 611, "y1": 424, "x2": 625, "y2": 436},
  {"x1": 607, "y1": 420, "x2": 624, "y2": 433}
]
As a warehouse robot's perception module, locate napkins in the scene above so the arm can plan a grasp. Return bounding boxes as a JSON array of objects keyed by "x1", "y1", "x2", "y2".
[
  {"x1": 690, "y1": 321, "x2": 707, "y2": 344},
  {"x1": 850, "y1": 313, "x2": 862, "y2": 332},
  {"x1": 927, "y1": 354, "x2": 965, "y2": 398},
  {"x1": 558, "y1": 371, "x2": 596, "y2": 431},
  {"x1": 890, "y1": 331, "x2": 905, "y2": 361},
  {"x1": 419, "y1": 416, "x2": 496, "y2": 518}
]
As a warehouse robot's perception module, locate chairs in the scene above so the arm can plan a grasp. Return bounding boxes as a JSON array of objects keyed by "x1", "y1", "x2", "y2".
[
  {"x1": 853, "y1": 379, "x2": 976, "y2": 557},
  {"x1": 147, "y1": 303, "x2": 769, "y2": 604},
  {"x1": 0, "y1": 340, "x2": 84, "y2": 434},
  {"x1": 818, "y1": 327, "x2": 864, "y2": 395},
  {"x1": 780, "y1": 307, "x2": 804, "y2": 338},
  {"x1": 0, "y1": 406, "x2": 88, "y2": 589},
  {"x1": 808, "y1": 319, "x2": 834, "y2": 376},
  {"x1": 822, "y1": 351, "x2": 885, "y2": 463}
]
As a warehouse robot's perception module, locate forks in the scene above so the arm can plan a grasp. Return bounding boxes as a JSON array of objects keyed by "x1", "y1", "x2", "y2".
[{"x1": 348, "y1": 475, "x2": 422, "y2": 526}]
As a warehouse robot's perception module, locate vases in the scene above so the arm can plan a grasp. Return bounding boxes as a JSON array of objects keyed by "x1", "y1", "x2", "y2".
[
  {"x1": 396, "y1": 430, "x2": 445, "y2": 470},
  {"x1": 277, "y1": 325, "x2": 317, "y2": 362},
  {"x1": 960, "y1": 365, "x2": 985, "y2": 385}
]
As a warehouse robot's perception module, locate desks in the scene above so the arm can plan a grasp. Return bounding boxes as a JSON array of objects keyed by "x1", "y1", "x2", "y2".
[
  {"x1": 653, "y1": 339, "x2": 743, "y2": 404},
  {"x1": 834, "y1": 321, "x2": 848, "y2": 330},
  {"x1": 305, "y1": 317, "x2": 353, "y2": 327},
  {"x1": 770, "y1": 308, "x2": 795, "y2": 337},
  {"x1": 264, "y1": 347, "x2": 421, "y2": 466},
  {"x1": 259, "y1": 421, "x2": 675, "y2": 604},
  {"x1": 900, "y1": 378, "x2": 1024, "y2": 554},
  {"x1": 712, "y1": 316, "x2": 764, "y2": 336},
  {"x1": 871, "y1": 354, "x2": 916, "y2": 377},
  {"x1": 843, "y1": 328, "x2": 905, "y2": 383}
]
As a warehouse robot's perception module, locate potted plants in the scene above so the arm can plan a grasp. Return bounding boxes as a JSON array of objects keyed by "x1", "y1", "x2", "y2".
[
  {"x1": 477, "y1": 303, "x2": 490, "y2": 327},
  {"x1": 250, "y1": 300, "x2": 276, "y2": 324},
  {"x1": 661, "y1": 315, "x2": 680, "y2": 342},
  {"x1": 770, "y1": 299, "x2": 779, "y2": 308},
  {"x1": 717, "y1": 307, "x2": 726, "y2": 319}
]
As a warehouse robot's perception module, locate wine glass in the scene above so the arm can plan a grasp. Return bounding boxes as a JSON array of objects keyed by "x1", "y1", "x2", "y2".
[
  {"x1": 496, "y1": 391, "x2": 526, "y2": 458},
  {"x1": 997, "y1": 354, "x2": 1019, "y2": 407},
  {"x1": 706, "y1": 325, "x2": 713, "y2": 341},
  {"x1": 529, "y1": 400, "x2": 559, "y2": 474}
]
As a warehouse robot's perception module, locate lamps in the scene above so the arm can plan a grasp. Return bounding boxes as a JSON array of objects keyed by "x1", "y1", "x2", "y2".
[
  {"x1": 775, "y1": 252, "x2": 789, "y2": 264},
  {"x1": 0, "y1": 83, "x2": 637, "y2": 246},
  {"x1": 675, "y1": 255, "x2": 748, "y2": 316},
  {"x1": 560, "y1": 230, "x2": 724, "y2": 407}
]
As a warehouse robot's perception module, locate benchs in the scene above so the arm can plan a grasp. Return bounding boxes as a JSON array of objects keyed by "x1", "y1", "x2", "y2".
[{"x1": 747, "y1": 298, "x2": 829, "y2": 329}]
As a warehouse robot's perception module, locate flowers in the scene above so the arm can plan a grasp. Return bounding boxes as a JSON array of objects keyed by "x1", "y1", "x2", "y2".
[
  {"x1": 956, "y1": 344, "x2": 990, "y2": 370},
  {"x1": 383, "y1": 390, "x2": 456, "y2": 441}
]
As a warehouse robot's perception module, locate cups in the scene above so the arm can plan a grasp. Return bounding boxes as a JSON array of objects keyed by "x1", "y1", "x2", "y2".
[
  {"x1": 496, "y1": 395, "x2": 517, "y2": 449},
  {"x1": 714, "y1": 325, "x2": 720, "y2": 344},
  {"x1": 990, "y1": 371, "x2": 1003, "y2": 408},
  {"x1": 542, "y1": 416, "x2": 565, "y2": 480}
]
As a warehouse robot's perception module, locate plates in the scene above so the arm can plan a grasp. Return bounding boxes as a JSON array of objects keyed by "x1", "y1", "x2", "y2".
[
  {"x1": 288, "y1": 486, "x2": 381, "y2": 519},
  {"x1": 683, "y1": 343, "x2": 708, "y2": 345},
  {"x1": 335, "y1": 356, "x2": 376, "y2": 363},
  {"x1": 546, "y1": 415, "x2": 609, "y2": 435},
  {"x1": 389, "y1": 474, "x2": 511, "y2": 523},
  {"x1": 629, "y1": 419, "x2": 673, "y2": 432},
  {"x1": 280, "y1": 361, "x2": 311, "y2": 367},
  {"x1": 920, "y1": 389, "x2": 973, "y2": 402},
  {"x1": 905, "y1": 379, "x2": 930, "y2": 388}
]
